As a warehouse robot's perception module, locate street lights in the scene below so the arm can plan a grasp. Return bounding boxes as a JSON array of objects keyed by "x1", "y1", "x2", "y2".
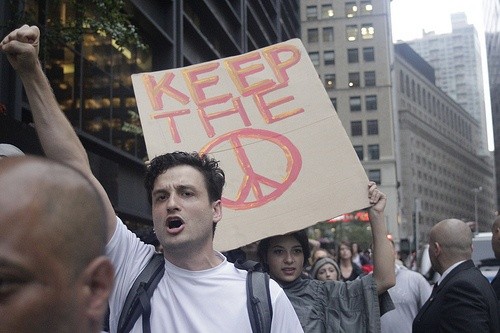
[{"x1": 472, "y1": 186, "x2": 483, "y2": 235}]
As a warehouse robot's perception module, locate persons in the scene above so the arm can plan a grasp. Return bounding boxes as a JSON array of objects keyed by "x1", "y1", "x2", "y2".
[
  {"x1": 304, "y1": 236, "x2": 419, "y2": 283},
  {"x1": 490, "y1": 218, "x2": 500, "y2": 308},
  {"x1": 359, "y1": 236, "x2": 435, "y2": 333},
  {"x1": 1, "y1": 24, "x2": 307, "y2": 333},
  {"x1": 247, "y1": 182, "x2": 398, "y2": 333},
  {"x1": 412, "y1": 219, "x2": 500, "y2": 333},
  {"x1": 0, "y1": 154, "x2": 116, "y2": 332},
  {"x1": 241, "y1": 239, "x2": 261, "y2": 261}
]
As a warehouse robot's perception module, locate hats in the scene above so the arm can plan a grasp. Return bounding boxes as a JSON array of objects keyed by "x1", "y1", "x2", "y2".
[{"x1": 311, "y1": 256, "x2": 342, "y2": 281}]
